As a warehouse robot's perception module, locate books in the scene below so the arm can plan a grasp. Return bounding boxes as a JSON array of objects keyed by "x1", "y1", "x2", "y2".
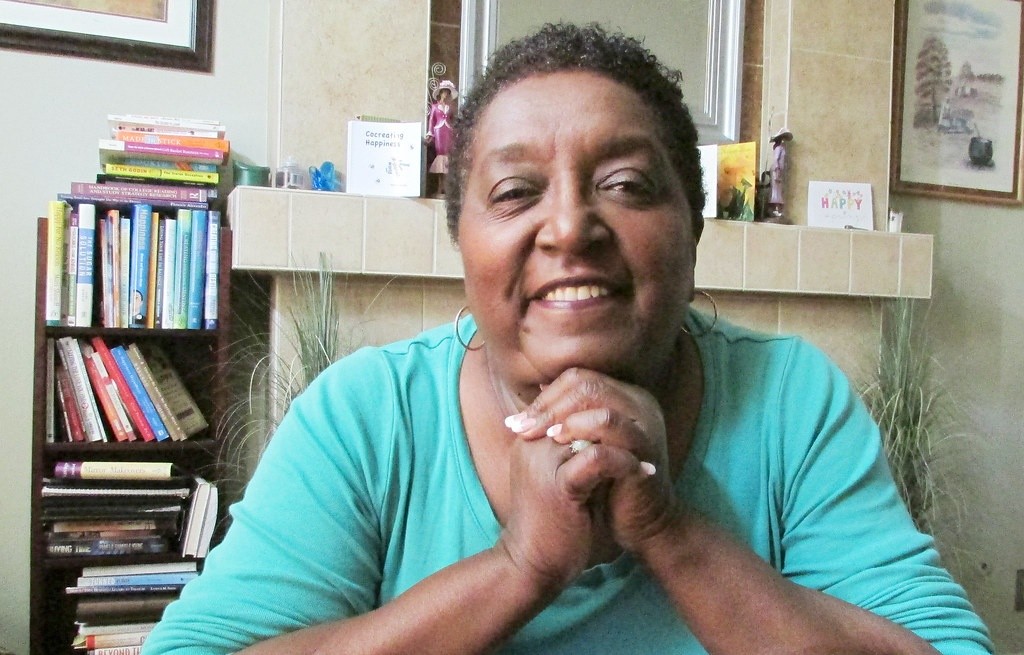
[
  {"x1": 695, "y1": 140, "x2": 875, "y2": 231},
  {"x1": 43, "y1": 113, "x2": 232, "y2": 331},
  {"x1": 346, "y1": 118, "x2": 422, "y2": 198},
  {"x1": 45, "y1": 338, "x2": 209, "y2": 444},
  {"x1": 41, "y1": 457, "x2": 217, "y2": 655}
]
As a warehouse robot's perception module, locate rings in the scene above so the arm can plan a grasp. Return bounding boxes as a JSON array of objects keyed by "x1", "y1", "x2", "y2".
[{"x1": 569, "y1": 438, "x2": 593, "y2": 453}]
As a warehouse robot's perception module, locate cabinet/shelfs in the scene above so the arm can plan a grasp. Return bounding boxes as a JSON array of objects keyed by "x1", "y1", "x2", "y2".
[{"x1": 30, "y1": 216, "x2": 231, "y2": 655}]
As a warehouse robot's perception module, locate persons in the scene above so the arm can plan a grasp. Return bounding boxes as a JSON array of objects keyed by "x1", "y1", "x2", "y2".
[
  {"x1": 139, "y1": 22, "x2": 995, "y2": 655},
  {"x1": 425, "y1": 80, "x2": 461, "y2": 200},
  {"x1": 767, "y1": 129, "x2": 794, "y2": 218}
]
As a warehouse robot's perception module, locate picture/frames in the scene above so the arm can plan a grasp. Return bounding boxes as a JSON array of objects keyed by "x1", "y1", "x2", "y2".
[
  {"x1": 0, "y1": 0, "x2": 217, "y2": 73},
  {"x1": 889, "y1": 0, "x2": 1024, "y2": 207},
  {"x1": 457, "y1": 0, "x2": 747, "y2": 147}
]
